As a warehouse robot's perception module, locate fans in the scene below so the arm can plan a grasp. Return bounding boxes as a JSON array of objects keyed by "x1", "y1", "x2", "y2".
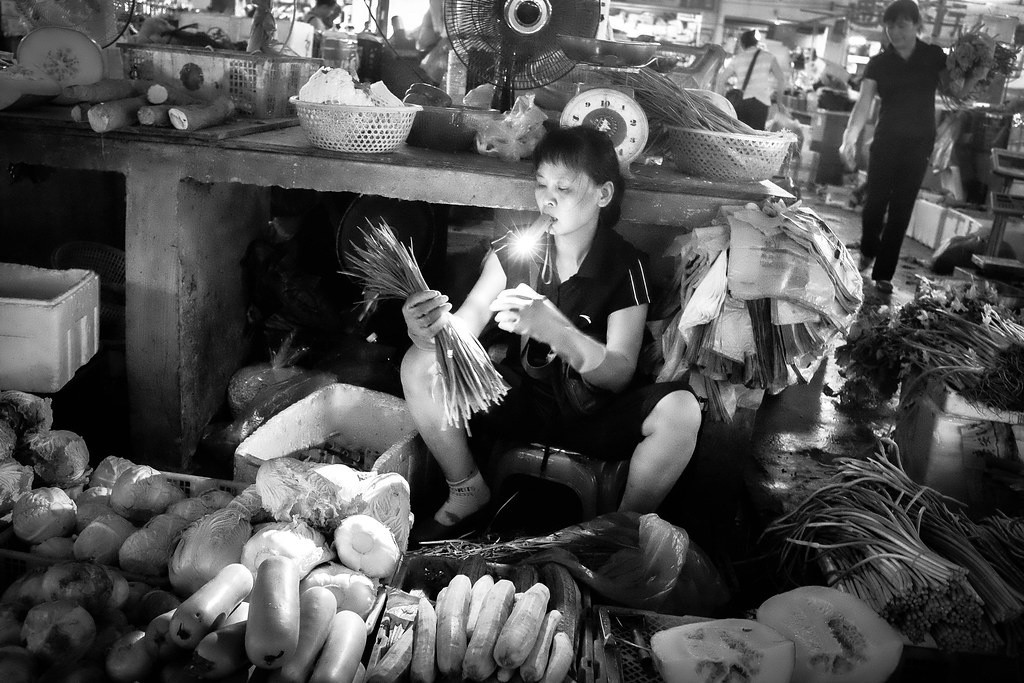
[
  {"x1": 444, "y1": 0, "x2": 601, "y2": 115},
  {"x1": 14, "y1": 0, "x2": 136, "y2": 49}
]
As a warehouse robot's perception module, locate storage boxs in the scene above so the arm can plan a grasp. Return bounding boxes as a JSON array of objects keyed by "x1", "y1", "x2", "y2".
[
  {"x1": 894, "y1": 362, "x2": 1024, "y2": 491},
  {"x1": 940, "y1": 208, "x2": 1024, "y2": 265},
  {"x1": 235, "y1": 383, "x2": 429, "y2": 485},
  {"x1": 913, "y1": 199, "x2": 948, "y2": 250},
  {"x1": 905, "y1": 189, "x2": 945, "y2": 238},
  {"x1": 594, "y1": 607, "x2": 717, "y2": 683},
  {"x1": 363, "y1": 553, "x2": 594, "y2": 683},
  {"x1": 798, "y1": 150, "x2": 844, "y2": 184},
  {"x1": 159, "y1": 472, "x2": 415, "y2": 634},
  {"x1": 0, "y1": 263, "x2": 100, "y2": 392},
  {"x1": 116, "y1": 43, "x2": 325, "y2": 118},
  {"x1": 811, "y1": 109, "x2": 865, "y2": 144},
  {"x1": 178, "y1": 13, "x2": 314, "y2": 59}
]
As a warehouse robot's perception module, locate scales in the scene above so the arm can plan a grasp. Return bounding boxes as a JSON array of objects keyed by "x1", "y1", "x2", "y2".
[{"x1": 555, "y1": 35, "x2": 661, "y2": 179}]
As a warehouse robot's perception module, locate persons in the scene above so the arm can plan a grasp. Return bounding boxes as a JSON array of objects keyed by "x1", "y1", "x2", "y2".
[
  {"x1": 838, "y1": 1, "x2": 996, "y2": 293},
  {"x1": 720, "y1": 30, "x2": 829, "y2": 131},
  {"x1": 304, "y1": 0, "x2": 356, "y2": 50},
  {"x1": 397, "y1": 125, "x2": 703, "y2": 542}
]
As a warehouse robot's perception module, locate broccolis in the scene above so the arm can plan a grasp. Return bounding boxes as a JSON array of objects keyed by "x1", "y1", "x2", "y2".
[{"x1": 297, "y1": 66, "x2": 357, "y2": 108}]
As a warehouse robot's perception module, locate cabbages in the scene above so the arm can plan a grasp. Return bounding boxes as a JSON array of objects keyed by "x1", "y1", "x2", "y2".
[{"x1": 0, "y1": 390, "x2": 411, "y2": 683}]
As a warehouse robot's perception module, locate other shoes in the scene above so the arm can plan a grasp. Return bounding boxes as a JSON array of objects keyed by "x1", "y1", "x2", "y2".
[
  {"x1": 412, "y1": 507, "x2": 488, "y2": 548},
  {"x1": 876, "y1": 280, "x2": 891, "y2": 292},
  {"x1": 860, "y1": 253, "x2": 872, "y2": 270}
]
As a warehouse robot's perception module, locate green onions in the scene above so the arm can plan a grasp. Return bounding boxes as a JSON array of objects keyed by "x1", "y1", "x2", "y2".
[{"x1": 616, "y1": 63, "x2": 792, "y2": 183}]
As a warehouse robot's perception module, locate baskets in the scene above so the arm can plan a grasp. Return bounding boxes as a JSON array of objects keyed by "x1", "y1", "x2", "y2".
[
  {"x1": 50, "y1": 240, "x2": 125, "y2": 346},
  {"x1": 667, "y1": 125, "x2": 798, "y2": 186},
  {"x1": 405, "y1": 104, "x2": 501, "y2": 152},
  {"x1": 289, "y1": 95, "x2": 423, "y2": 154},
  {"x1": 115, "y1": 43, "x2": 324, "y2": 121}
]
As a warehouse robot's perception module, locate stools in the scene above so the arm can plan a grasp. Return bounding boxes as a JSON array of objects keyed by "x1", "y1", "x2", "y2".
[{"x1": 484, "y1": 434, "x2": 630, "y2": 521}]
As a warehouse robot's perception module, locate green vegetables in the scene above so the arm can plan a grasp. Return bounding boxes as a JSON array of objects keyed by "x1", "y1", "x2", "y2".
[{"x1": 819, "y1": 272, "x2": 1024, "y2": 414}]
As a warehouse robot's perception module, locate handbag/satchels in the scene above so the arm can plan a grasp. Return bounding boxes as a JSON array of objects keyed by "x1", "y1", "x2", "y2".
[
  {"x1": 546, "y1": 351, "x2": 616, "y2": 419},
  {"x1": 726, "y1": 89, "x2": 743, "y2": 113}
]
